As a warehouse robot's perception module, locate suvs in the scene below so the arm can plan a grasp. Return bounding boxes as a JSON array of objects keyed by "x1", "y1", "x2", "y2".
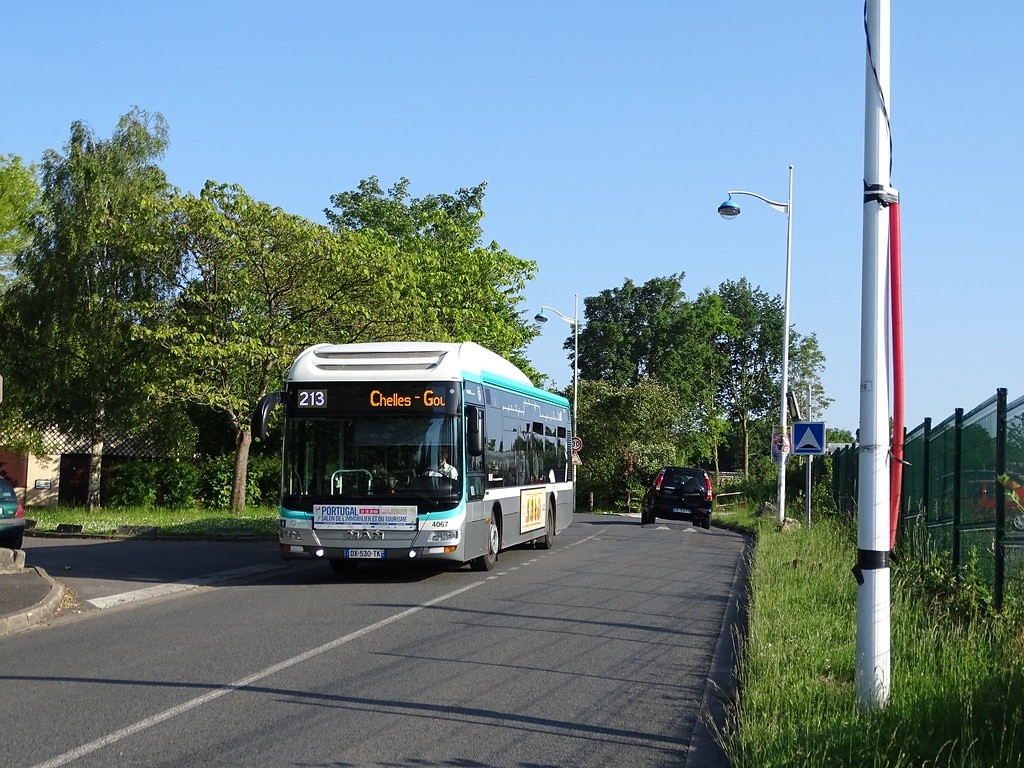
[{"x1": 640, "y1": 465, "x2": 712, "y2": 530}]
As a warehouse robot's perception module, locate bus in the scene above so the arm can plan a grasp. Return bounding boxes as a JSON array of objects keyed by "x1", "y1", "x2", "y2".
[{"x1": 251, "y1": 340, "x2": 575, "y2": 576}]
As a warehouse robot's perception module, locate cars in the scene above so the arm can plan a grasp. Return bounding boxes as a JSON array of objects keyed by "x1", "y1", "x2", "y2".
[
  {"x1": 0, "y1": 475, "x2": 27, "y2": 550},
  {"x1": 939, "y1": 474, "x2": 1024, "y2": 518}
]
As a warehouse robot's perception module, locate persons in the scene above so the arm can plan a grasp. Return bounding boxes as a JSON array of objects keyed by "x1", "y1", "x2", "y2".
[
  {"x1": 419, "y1": 447, "x2": 459, "y2": 490},
  {"x1": 363, "y1": 448, "x2": 405, "y2": 486},
  {"x1": 504, "y1": 457, "x2": 515, "y2": 486}
]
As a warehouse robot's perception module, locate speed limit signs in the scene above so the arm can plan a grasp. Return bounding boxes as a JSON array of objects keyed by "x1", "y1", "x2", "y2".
[{"x1": 572, "y1": 436, "x2": 583, "y2": 451}]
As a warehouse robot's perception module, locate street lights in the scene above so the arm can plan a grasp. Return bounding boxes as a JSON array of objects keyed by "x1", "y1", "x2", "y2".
[
  {"x1": 717, "y1": 165, "x2": 795, "y2": 527},
  {"x1": 534, "y1": 293, "x2": 579, "y2": 514}
]
